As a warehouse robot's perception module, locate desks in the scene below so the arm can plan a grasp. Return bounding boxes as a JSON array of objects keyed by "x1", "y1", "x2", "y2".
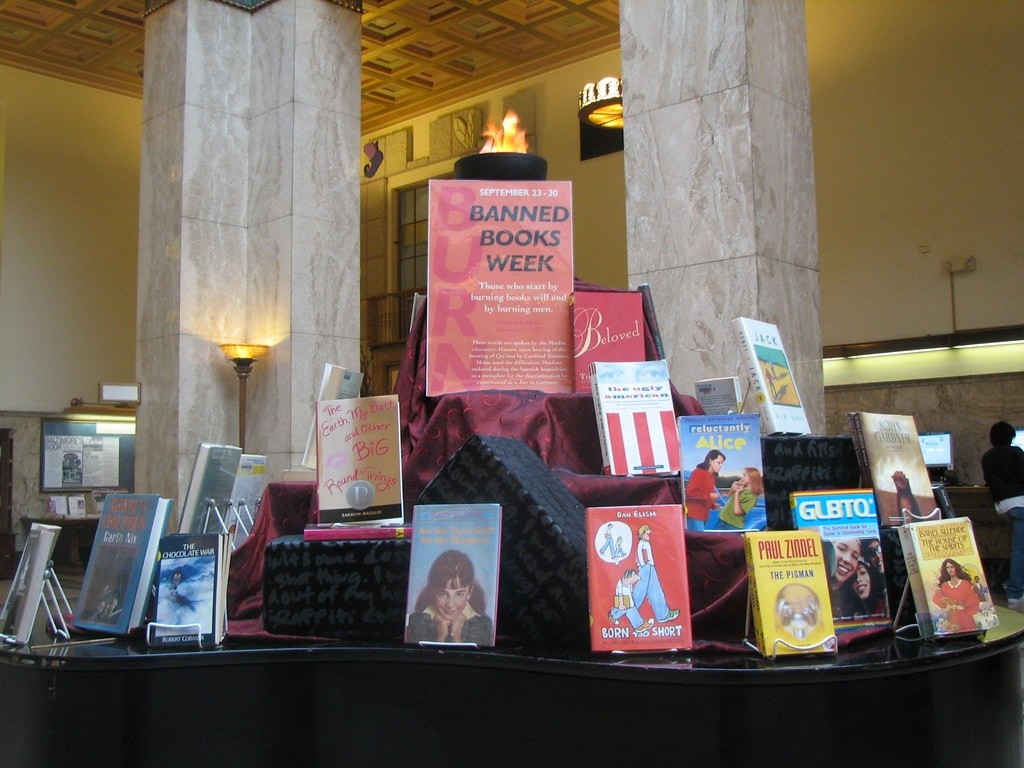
[
  {"x1": 22, "y1": 516, "x2": 101, "y2": 570},
  {"x1": 945, "y1": 486, "x2": 995, "y2": 512}
]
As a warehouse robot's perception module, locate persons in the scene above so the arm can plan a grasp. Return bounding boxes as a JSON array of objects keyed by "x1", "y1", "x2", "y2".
[
  {"x1": 826, "y1": 538, "x2": 886, "y2": 617},
  {"x1": 981, "y1": 420, "x2": 1024, "y2": 602},
  {"x1": 685, "y1": 449, "x2": 764, "y2": 530},
  {"x1": 407, "y1": 550, "x2": 493, "y2": 645},
  {"x1": 973, "y1": 575, "x2": 986, "y2": 603},
  {"x1": 173, "y1": 569, "x2": 181, "y2": 586},
  {"x1": 933, "y1": 558, "x2": 980, "y2": 632}
]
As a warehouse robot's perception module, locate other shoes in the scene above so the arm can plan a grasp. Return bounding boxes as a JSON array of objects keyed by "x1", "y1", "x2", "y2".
[{"x1": 1008, "y1": 593, "x2": 1024, "y2": 604}]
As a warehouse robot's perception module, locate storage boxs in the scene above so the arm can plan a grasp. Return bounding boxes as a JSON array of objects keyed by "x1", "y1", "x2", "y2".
[
  {"x1": 264, "y1": 535, "x2": 411, "y2": 640},
  {"x1": 418, "y1": 434, "x2": 590, "y2": 650},
  {"x1": 762, "y1": 432, "x2": 860, "y2": 531}
]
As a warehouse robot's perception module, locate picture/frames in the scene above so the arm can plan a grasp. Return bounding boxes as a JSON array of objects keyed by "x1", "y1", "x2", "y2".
[{"x1": 40, "y1": 416, "x2": 136, "y2": 494}]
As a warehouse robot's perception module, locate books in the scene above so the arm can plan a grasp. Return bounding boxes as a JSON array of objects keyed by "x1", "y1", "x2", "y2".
[
  {"x1": 402, "y1": 504, "x2": 503, "y2": 647},
  {"x1": 695, "y1": 376, "x2": 745, "y2": 414},
  {"x1": 589, "y1": 360, "x2": 681, "y2": 477},
  {"x1": 848, "y1": 411, "x2": 956, "y2": 525},
  {"x1": 789, "y1": 488, "x2": 893, "y2": 633},
  {"x1": 585, "y1": 504, "x2": 693, "y2": 652},
  {"x1": 301, "y1": 363, "x2": 405, "y2": 527},
  {"x1": 569, "y1": 291, "x2": 645, "y2": 393},
  {"x1": 0, "y1": 522, "x2": 62, "y2": 643},
  {"x1": 731, "y1": 317, "x2": 813, "y2": 435},
  {"x1": 897, "y1": 516, "x2": 999, "y2": 638},
  {"x1": 638, "y1": 284, "x2": 667, "y2": 359},
  {"x1": 742, "y1": 530, "x2": 839, "y2": 657},
  {"x1": 145, "y1": 442, "x2": 270, "y2": 649},
  {"x1": 73, "y1": 493, "x2": 174, "y2": 636},
  {"x1": 677, "y1": 412, "x2": 768, "y2": 533}
]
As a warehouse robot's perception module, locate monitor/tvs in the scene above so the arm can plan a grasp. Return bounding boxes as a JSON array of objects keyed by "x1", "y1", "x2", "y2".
[
  {"x1": 917, "y1": 431, "x2": 953, "y2": 467},
  {"x1": 1010, "y1": 427, "x2": 1024, "y2": 451}
]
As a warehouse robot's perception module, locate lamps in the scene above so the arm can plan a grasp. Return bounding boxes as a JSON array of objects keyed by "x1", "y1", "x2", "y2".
[
  {"x1": 940, "y1": 254, "x2": 976, "y2": 274},
  {"x1": 578, "y1": 78, "x2": 623, "y2": 128},
  {"x1": 218, "y1": 344, "x2": 270, "y2": 453}
]
